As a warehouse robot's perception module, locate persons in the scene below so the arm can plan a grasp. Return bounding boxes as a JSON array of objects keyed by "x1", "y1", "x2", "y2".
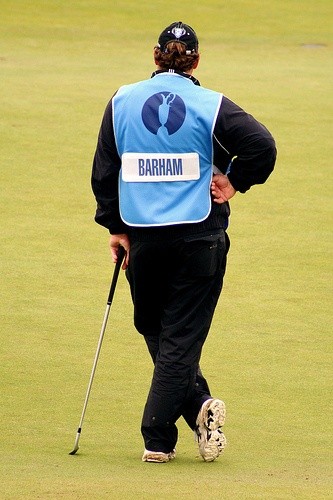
[{"x1": 91, "y1": 20, "x2": 277, "y2": 463}]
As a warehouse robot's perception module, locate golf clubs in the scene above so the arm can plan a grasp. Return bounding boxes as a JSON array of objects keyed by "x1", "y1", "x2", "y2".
[{"x1": 68, "y1": 244, "x2": 125, "y2": 456}]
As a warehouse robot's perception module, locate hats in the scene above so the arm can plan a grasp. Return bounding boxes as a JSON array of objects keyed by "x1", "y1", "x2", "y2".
[{"x1": 158, "y1": 22, "x2": 198, "y2": 50}]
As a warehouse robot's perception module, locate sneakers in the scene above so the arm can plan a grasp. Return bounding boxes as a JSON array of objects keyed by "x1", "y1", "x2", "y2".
[
  {"x1": 142, "y1": 449, "x2": 176, "y2": 462},
  {"x1": 194, "y1": 398, "x2": 227, "y2": 462}
]
